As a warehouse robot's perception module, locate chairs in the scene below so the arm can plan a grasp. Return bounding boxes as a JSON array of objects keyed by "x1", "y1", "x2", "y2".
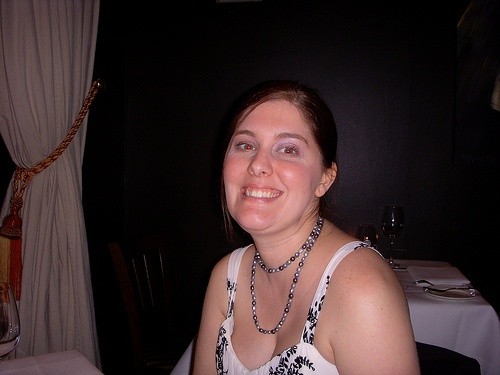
[{"x1": 106, "y1": 210, "x2": 235, "y2": 371}]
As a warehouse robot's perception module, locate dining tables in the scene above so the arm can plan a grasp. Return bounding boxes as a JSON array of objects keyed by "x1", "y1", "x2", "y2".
[
  {"x1": 0, "y1": 349, "x2": 105, "y2": 375},
  {"x1": 387, "y1": 258, "x2": 500, "y2": 375}
]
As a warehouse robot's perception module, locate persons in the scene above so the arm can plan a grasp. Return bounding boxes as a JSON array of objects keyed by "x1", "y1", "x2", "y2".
[{"x1": 192, "y1": 79, "x2": 420, "y2": 375}]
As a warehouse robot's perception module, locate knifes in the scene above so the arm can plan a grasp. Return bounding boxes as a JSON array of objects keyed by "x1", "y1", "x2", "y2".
[{"x1": 424, "y1": 287, "x2": 475, "y2": 290}]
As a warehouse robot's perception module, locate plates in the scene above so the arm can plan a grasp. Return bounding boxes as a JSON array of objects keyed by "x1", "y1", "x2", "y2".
[{"x1": 425, "y1": 289, "x2": 471, "y2": 299}]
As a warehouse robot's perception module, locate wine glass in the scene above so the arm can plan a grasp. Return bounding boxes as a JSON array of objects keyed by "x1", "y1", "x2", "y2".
[{"x1": 381, "y1": 205, "x2": 408, "y2": 269}]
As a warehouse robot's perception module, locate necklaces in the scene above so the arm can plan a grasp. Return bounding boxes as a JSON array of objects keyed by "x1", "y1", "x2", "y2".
[{"x1": 250, "y1": 218, "x2": 323, "y2": 335}]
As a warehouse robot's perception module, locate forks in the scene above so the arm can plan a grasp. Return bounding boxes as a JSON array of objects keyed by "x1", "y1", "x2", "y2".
[{"x1": 408, "y1": 280, "x2": 468, "y2": 286}]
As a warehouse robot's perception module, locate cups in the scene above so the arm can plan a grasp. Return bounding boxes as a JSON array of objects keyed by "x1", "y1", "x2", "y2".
[
  {"x1": 358, "y1": 225, "x2": 377, "y2": 246},
  {"x1": 0, "y1": 282, "x2": 21, "y2": 358}
]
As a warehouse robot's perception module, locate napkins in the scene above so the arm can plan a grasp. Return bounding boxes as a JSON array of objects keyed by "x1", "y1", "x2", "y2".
[{"x1": 406, "y1": 266, "x2": 471, "y2": 287}]
